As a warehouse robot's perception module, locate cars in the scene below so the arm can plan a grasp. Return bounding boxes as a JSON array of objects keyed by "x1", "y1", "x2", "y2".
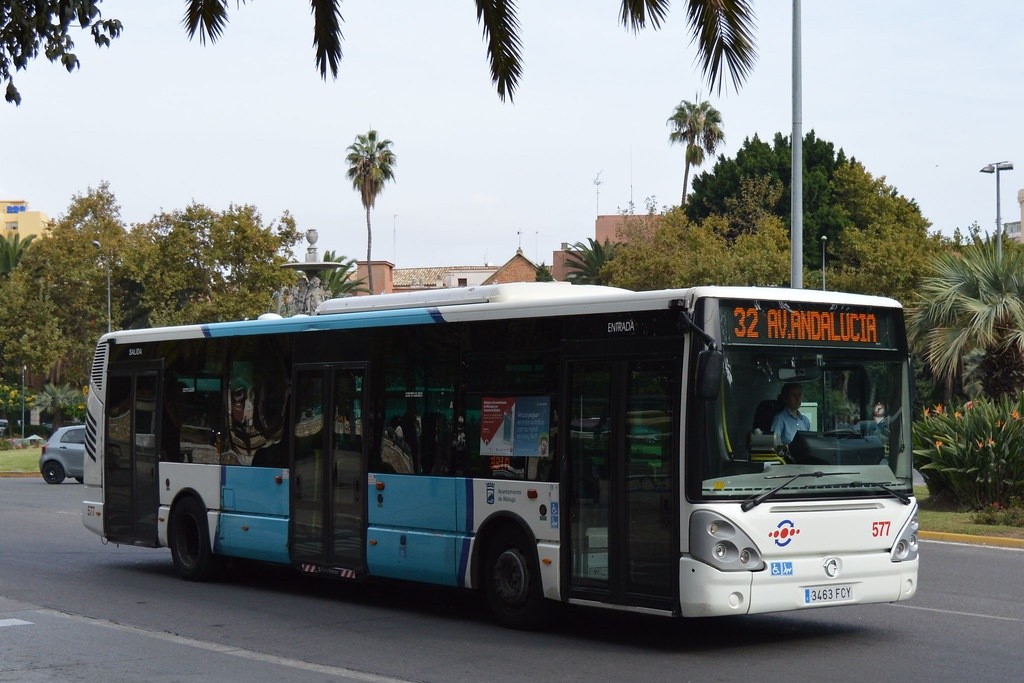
[
  {"x1": 39, "y1": 425, "x2": 86, "y2": 484},
  {"x1": 0, "y1": 419, "x2": 21, "y2": 436}
]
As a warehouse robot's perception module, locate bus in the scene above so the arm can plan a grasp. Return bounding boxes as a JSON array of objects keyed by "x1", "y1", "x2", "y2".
[{"x1": 82, "y1": 281, "x2": 919, "y2": 630}]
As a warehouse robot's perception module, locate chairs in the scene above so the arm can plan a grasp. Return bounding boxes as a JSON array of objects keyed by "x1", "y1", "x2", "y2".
[{"x1": 752, "y1": 400, "x2": 784, "y2": 434}]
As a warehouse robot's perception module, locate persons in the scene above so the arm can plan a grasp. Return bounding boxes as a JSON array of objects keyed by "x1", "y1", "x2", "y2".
[
  {"x1": 290, "y1": 277, "x2": 333, "y2": 314},
  {"x1": 769, "y1": 383, "x2": 810, "y2": 464}
]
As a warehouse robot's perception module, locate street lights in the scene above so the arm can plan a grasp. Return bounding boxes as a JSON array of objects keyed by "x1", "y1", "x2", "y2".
[
  {"x1": 980, "y1": 161, "x2": 1013, "y2": 263},
  {"x1": 93, "y1": 241, "x2": 110, "y2": 333},
  {"x1": 821, "y1": 236, "x2": 827, "y2": 290}
]
